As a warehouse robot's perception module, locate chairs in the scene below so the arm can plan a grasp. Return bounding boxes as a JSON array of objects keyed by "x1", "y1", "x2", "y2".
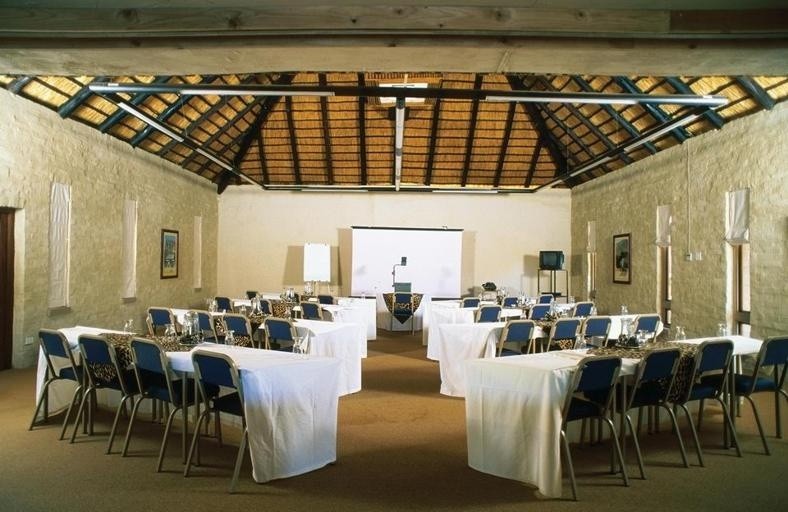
[{"x1": 390, "y1": 292, "x2": 414, "y2": 336}]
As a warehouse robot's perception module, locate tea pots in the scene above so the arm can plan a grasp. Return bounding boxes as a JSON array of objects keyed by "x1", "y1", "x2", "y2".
[{"x1": 181, "y1": 311, "x2": 200, "y2": 340}]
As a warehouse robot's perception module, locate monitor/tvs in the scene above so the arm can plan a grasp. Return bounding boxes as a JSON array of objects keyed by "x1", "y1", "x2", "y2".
[{"x1": 539, "y1": 251, "x2": 564, "y2": 269}]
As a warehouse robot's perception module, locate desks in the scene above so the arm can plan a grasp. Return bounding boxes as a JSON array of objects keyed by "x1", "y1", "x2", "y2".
[{"x1": 376, "y1": 292, "x2": 433, "y2": 331}]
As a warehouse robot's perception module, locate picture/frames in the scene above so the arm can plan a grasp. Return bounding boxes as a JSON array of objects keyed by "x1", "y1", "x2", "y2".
[
  {"x1": 161, "y1": 228, "x2": 179, "y2": 279},
  {"x1": 612, "y1": 233, "x2": 632, "y2": 284}
]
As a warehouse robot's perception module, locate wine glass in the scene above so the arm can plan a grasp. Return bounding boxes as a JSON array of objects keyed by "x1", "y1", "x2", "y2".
[
  {"x1": 164, "y1": 323, "x2": 178, "y2": 340},
  {"x1": 210, "y1": 286, "x2": 366, "y2": 324},
  {"x1": 717, "y1": 324, "x2": 729, "y2": 338},
  {"x1": 574, "y1": 333, "x2": 587, "y2": 354},
  {"x1": 638, "y1": 330, "x2": 649, "y2": 348},
  {"x1": 291, "y1": 336, "x2": 304, "y2": 361},
  {"x1": 674, "y1": 325, "x2": 687, "y2": 343},
  {"x1": 123, "y1": 319, "x2": 135, "y2": 337},
  {"x1": 224, "y1": 329, "x2": 235, "y2": 354},
  {"x1": 497, "y1": 290, "x2": 629, "y2": 317}
]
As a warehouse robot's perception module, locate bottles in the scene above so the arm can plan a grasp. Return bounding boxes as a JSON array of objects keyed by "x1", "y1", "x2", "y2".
[{"x1": 619, "y1": 318, "x2": 634, "y2": 339}]
